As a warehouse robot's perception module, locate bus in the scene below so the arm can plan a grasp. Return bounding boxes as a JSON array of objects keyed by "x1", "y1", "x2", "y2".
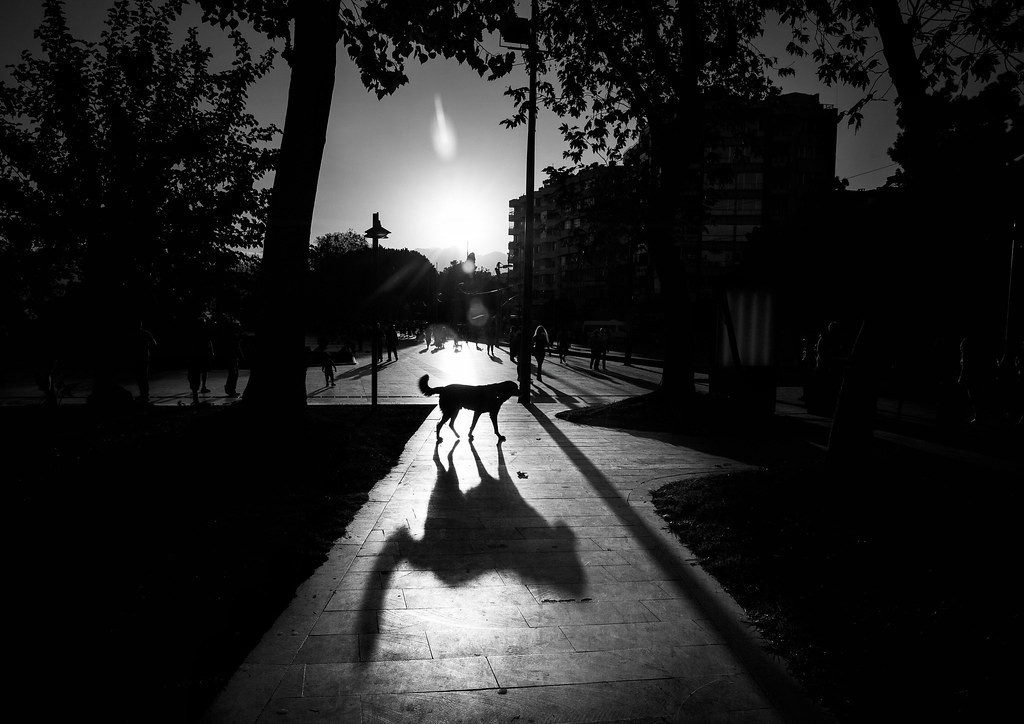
[{"x1": 582, "y1": 320, "x2": 626, "y2": 341}]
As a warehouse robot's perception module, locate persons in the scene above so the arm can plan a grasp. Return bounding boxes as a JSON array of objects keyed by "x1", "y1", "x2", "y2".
[
  {"x1": 322, "y1": 352, "x2": 336, "y2": 386},
  {"x1": 509, "y1": 325, "x2": 532, "y2": 381},
  {"x1": 533, "y1": 325, "x2": 551, "y2": 381},
  {"x1": 187, "y1": 313, "x2": 244, "y2": 395},
  {"x1": 377, "y1": 322, "x2": 384, "y2": 362},
  {"x1": 387, "y1": 325, "x2": 398, "y2": 361},
  {"x1": 556, "y1": 331, "x2": 568, "y2": 362},
  {"x1": 798, "y1": 322, "x2": 836, "y2": 400},
  {"x1": 486, "y1": 324, "x2": 496, "y2": 355},
  {"x1": 426, "y1": 327, "x2": 446, "y2": 348},
  {"x1": 589, "y1": 328, "x2": 609, "y2": 370}
]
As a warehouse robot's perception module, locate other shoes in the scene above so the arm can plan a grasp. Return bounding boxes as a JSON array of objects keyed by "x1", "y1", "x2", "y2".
[
  {"x1": 331, "y1": 384, "x2": 336, "y2": 386},
  {"x1": 201, "y1": 388, "x2": 211, "y2": 393}
]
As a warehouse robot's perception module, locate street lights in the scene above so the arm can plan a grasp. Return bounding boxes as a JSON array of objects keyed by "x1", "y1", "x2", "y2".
[
  {"x1": 501, "y1": 0, "x2": 539, "y2": 403},
  {"x1": 495, "y1": 262, "x2": 512, "y2": 347},
  {"x1": 366, "y1": 213, "x2": 391, "y2": 405}
]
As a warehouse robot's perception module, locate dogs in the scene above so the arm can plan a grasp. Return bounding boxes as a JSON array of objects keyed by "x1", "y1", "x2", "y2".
[{"x1": 417, "y1": 374, "x2": 523, "y2": 440}]
